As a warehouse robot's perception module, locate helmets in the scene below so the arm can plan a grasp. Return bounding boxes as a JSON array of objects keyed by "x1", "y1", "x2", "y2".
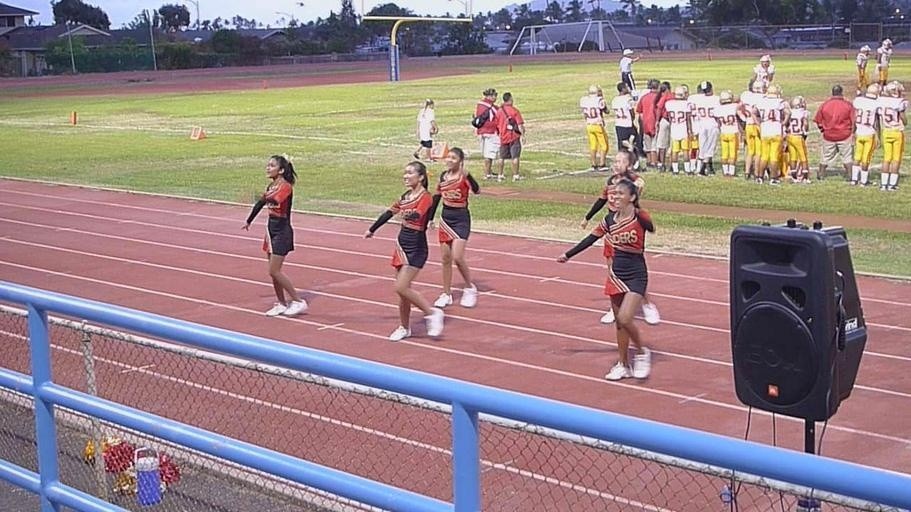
[
  {"x1": 860, "y1": 45, "x2": 871, "y2": 53},
  {"x1": 766, "y1": 84, "x2": 782, "y2": 99},
  {"x1": 623, "y1": 48, "x2": 635, "y2": 56},
  {"x1": 790, "y1": 95, "x2": 806, "y2": 110},
  {"x1": 719, "y1": 89, "x2": 733, "y2": 105},
  {"x1": 864, "y1": 83, "x2": 882, "y2": 99},
  {"x1": 752, "y1": 79, "x2": 767, "y2": 94},
  {"x1": 882, "y1": 39, "x2": 892, "y2": 50},
  {"x1": 589, "y1": 85, "x2": 603, "y2": 97},
  {"x1": 884, "y1": 80, "x2": 905, "y2": 98},
  {"x1": 759, "y1": 54, "x2": 771, "y2": 63}
]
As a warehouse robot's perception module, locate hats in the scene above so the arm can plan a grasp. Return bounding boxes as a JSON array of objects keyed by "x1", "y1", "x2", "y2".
[
  {"x1": 696, "y1": 81, "x2": 713, "y2": 92},
  {"x1": 674, "y1": 83, "x2": 689, "y2": 101}
]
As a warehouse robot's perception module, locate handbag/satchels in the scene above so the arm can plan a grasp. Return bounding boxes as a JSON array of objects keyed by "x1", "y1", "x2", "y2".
[
  {"x1": 471, "y1": 104, "x2": 494, "y2": 130},
  {"x1": 508, "y1": 118, "x2": 522, "y2": 134}
]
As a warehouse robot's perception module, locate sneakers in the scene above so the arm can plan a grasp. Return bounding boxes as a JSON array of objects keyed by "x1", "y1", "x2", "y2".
[
  {"x1": 483, "y1": 172, "x2": 497, "y2": 181},
  {"x1": 459, "y1": 282, "x2": 478, "y2": 308},
  {"x1": 590, "y1": 165, "x2": 610, "y2": 172},
  {"x1": 264, "y1": 303, "x2": 289, "y2": 316},
  {"x1": 600, "y1": 306, "x2": 616, "y2": 324},
  {"x1": 720, "y1": 165, "x2": 811, "y2": 185},
  {"x1": 423, "y1": 308, "x2": 445, "y2": 337},
  {"x1": 282, "y1": 299, "x2": 309, "y2": 316},
  {"x1": 641, "y1": 301, "x2": 660, "y2": 325},
  {"x1": 605, "y1": 362, "x2": 633, "y2": 381},
  {"x1": 816, "y1": 176, "x2": 899, "y2": 192},
  {"x1": 497, "y1": 173, "x2": 507, "y2": 183},
  {"x1": 388, "y1": 325, "x2": 412, "y2": 342},
  {"x1": 434, "y1": 293, "x2": 454, "y2": 307},
  {"x1": 633, "y1": 346, "x2": 652, "y2": 378},
  {"x1": 630, "y1": 162, "x2": 715, "y2": 176},
  {"x1": 511, "y1": 174, "x2": 528, "y2": 182}
]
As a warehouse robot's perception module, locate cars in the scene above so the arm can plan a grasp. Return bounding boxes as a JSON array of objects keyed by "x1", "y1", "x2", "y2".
[{"x1": 354, "y1": 16, "x2": 560, "y2": 54}]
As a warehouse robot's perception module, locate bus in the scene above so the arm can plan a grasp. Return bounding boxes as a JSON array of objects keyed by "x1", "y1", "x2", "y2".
[{"x1": 781, "y1": 26, "x2": 851, "y2": 47}]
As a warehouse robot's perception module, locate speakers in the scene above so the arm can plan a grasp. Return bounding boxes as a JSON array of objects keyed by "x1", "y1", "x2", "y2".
[{"x1": 730, "y1": 219, "x2": 867, "y2": 422}]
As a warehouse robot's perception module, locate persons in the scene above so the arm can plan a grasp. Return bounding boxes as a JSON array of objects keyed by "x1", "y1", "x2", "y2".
[
  {"x1": 557, "y1": 180, "x2": 656, "y2": 381},
  {"x1": 476, "y1": 89, "x2": 499, "y2": 178},
  {"x1": 239, "y1": 155, "x2": 309, "y2": 318},
  {"x1": 412, "y1": 99, "x2": 440, "y2": 164},
  {"x1": 580, "y1": 149, "x2": 662, "y2": 326},
  {"x1": 430, "y1": 147, "x2": 481, "y2": 309},
  {"x1": 495, "y1": 92, "x2": 526, "y2": 183},
  {"x1": 363, "y1": 161, "x2": 445, "y2": 344}
]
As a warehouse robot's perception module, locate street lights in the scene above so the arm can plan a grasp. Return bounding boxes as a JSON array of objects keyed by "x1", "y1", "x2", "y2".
[
  {"x1": 64, "y1": 14, "x2": 82, "y2": 74},
  {"x1": 274, "y1": 10, "x2": 296, "y2": 58},
  {"x1": 892, "y1": 7, "x2": 906, "y2": 45},
  {"x1": 449, "y1": 0, "x2": 468, "y2": 19},
  {"x1": 146, "y1": 8, "x2": 164, "y2": 71}
]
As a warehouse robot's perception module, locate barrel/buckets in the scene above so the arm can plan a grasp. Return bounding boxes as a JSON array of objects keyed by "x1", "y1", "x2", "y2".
[{"x1": 134, "y1": 446, "x2": 162, "y2": 506}]
